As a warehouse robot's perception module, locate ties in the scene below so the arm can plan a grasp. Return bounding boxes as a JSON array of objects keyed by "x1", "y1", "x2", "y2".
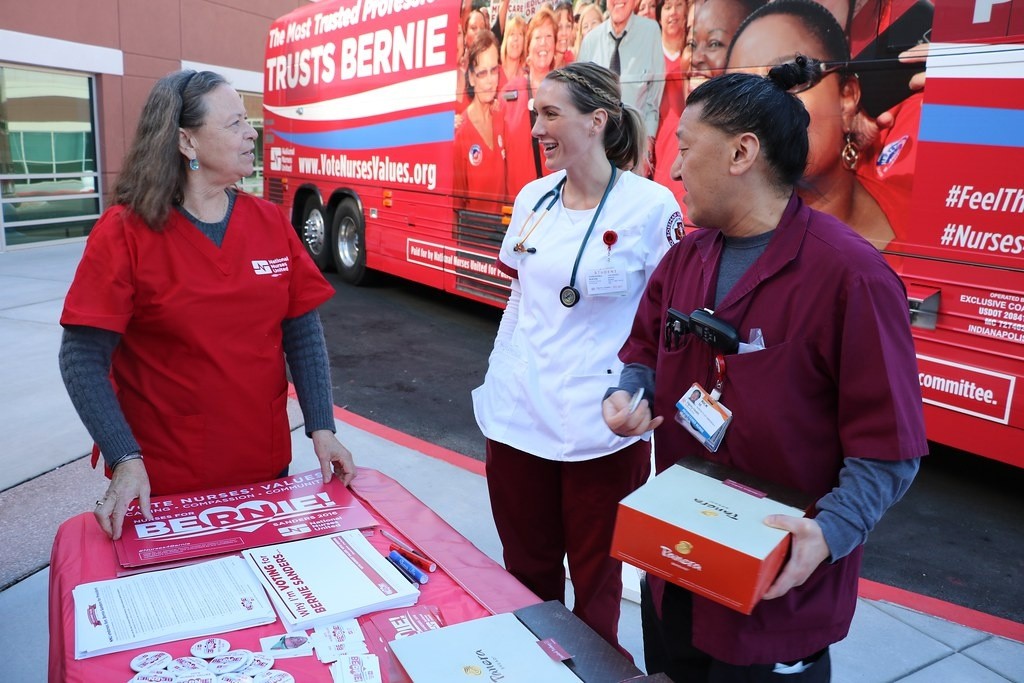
[{"x1": 609, "y1": 30, "x2": 627, "y2": 76}]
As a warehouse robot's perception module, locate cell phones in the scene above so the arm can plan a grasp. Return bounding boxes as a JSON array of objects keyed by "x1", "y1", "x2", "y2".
[{"x1": 847, "y1": 0, "x2": 935, "y2": 118}]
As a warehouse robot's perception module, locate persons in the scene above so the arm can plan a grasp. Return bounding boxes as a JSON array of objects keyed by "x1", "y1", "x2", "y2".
[
  {"x1": 689, "y1": 389, "x2": 701, "y2": 404},
  {"x1": 56, "y1": 70, "x2": 357, "y2": 540},
  {"x1": 601, "y1": 55, "x2": 930, "y2": 683},
  {"x1": 470, "y1": 61, "x2": 687, "y2": 661},
  {"x1": 453, "y1": 0, "x2": 935, "y2": 266}
]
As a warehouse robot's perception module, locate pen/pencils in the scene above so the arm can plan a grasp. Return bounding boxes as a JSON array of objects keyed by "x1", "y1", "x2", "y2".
[
  {"x1": 665, "y1": 322, "x2": 673, "y2": 351},
  {"x1": 671, "y1": 321, "x2": 682, "y2": 350},
  {"x1": 380, "y1": 529, "x2": 424, "y2": 558}
]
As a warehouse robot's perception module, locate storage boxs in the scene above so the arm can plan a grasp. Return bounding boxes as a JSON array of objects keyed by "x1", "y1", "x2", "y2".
[{"x1": 609, "y1": 460, "x2": 816, "y2": 619}]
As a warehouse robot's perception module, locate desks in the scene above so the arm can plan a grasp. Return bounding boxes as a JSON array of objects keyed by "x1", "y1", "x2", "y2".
[{"x1": 45, "y1": 461, "x2": 676, "y2": 683}]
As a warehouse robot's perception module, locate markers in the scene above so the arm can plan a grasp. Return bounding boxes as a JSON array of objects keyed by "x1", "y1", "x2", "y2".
[
  {"x1": 389, "y1": 544, "x2": 437, "y2": 573},
  {"x1": 389, "y1": 550, "x2": 429, "y2": 584},
  {"x1": 384, "y1": 557, "x2": 420, "y2": 589}
]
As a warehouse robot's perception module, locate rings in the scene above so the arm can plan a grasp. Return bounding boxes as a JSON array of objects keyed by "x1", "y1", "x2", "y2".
[{"x1": 96, "y1": 501, "x2": 103, "y2": 505}]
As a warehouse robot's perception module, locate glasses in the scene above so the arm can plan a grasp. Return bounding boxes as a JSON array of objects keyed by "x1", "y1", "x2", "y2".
[
  {"x1": 472, "y1": 63, "x2": 501, "y2": 76},
  {"x1": 784, "y1": 59, "x2": 851, "y2": 95}
]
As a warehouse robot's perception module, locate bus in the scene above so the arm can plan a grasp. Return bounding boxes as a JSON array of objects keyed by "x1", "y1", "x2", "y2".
[{"x1": 262, "y1": 0, "x2": 1024, "y2": 470}]
